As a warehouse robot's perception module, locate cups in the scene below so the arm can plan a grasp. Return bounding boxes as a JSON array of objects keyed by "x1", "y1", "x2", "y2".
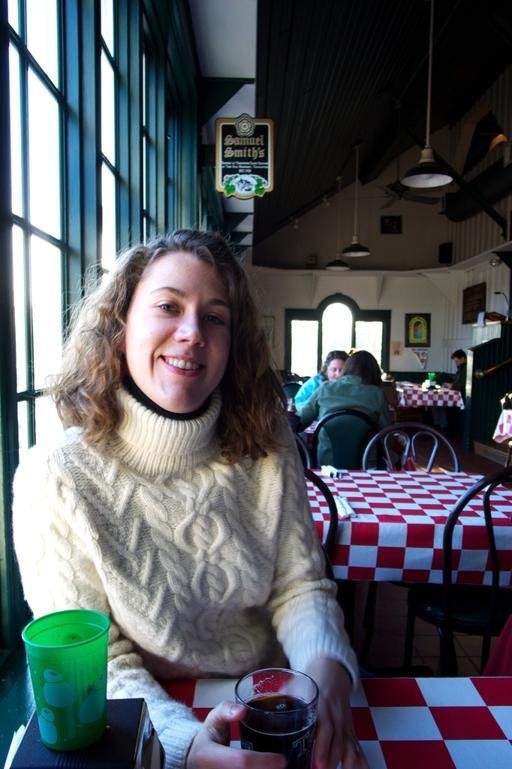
[
  {"x1": 21, "y1": 610, "x2": 111, "y2": 751},
  {"x1": 234, "y1": 667, "x2": 319, "y2": 769}
]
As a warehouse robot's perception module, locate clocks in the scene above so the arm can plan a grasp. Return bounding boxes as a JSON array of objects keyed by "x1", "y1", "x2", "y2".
[{"x1": 306, "y1": 254, "x2": 317, "y2": 265}]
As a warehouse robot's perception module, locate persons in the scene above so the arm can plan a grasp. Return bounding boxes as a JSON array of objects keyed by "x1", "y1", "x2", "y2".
[
  {"x1": 9, "y1": 229, "x2": 371, "y2": 768},
  {"x1": 297, "y1": 350, "x2": 388, "y2": 471},
  {"x1": 293, "y1": 350, "x2": 348, "y2": 417},
  {"x1": 444, "y1": 350, "x2": 467, "y2": 393}
]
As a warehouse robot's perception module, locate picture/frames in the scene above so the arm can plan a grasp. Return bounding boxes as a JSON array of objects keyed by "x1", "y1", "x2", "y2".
[
  {"x1": 438, "y1": 241, "x2": 453, "y2": 263},
  {"x1": 405, "y1": 312, "x2": 431, "y2": 347},
  {"x1": 263, "y1": 315, "x2": 277, "y2": 347},
  {"x1": 462, "y1": 282, "x2": 487, "y2": 324},
  {"x1": 381, "y1": 215, "x2": 402, "y2": 234}
]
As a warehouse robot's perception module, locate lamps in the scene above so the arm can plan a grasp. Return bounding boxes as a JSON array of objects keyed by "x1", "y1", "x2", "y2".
[
  {"x1": 490, "y1": 258, "x2": 500, "y2": 266},
  {"x1": 343, "y1": 142, "x2": 370, "y2": 257},
  {"x1": 401, "y1": 0, "x2": 454, "y2": 188},
  {"x1": 321, "y1": 194, "x2": 330, "y2": 205},
  {"x1": 290, "y1": 216, "x2": 300, "y2": 227},
  {"x1": 325, "y1": 176, "x2": 351, "y2": 270}
]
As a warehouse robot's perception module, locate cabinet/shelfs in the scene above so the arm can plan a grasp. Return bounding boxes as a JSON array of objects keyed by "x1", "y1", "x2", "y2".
[{"x1": 472, "y1": 320, "x2": 512, "y2": 345}]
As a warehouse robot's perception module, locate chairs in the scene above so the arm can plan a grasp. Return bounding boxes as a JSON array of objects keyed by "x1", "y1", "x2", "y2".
[
  {"x1": 363, "y1": 421, "x2": 459, "y2": 655},
  {"x1": 303, "y1": 465, "x2": 339, "y2": 581},
  {"x1": 282, "y1": 383, "x2": 300, "y2": 397},
  {"x1": 310, "y1": 408, "x2": 386, "y2": 469},
  {"x1": 292, "y1": 429, "x2": 310, "y2": 470},
  {"x1": 403, "y1": 466, "x2": 512, "y2": 676}
]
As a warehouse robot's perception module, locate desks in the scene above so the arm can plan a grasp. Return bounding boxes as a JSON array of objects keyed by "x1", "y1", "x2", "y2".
[{"x1": 504, "y1": 409, "x2": 512, "y2": 466}]
